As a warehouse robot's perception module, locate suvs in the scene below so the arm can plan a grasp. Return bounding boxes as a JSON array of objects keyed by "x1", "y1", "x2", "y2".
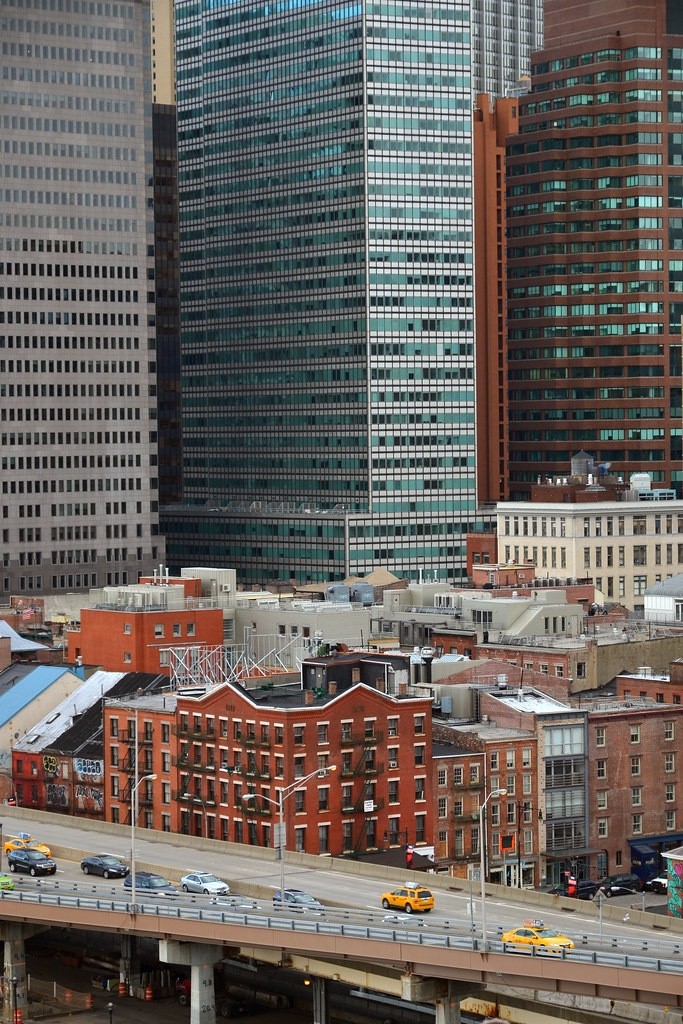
[
  {"x1": 547, "y1": 877, "x2": 601, "y2": 900},
  {"x1": 381, "y1": 881, "x2": 435, "y2": 914},
  {"x1": 598, "y1": 873, "x2": 645, "y2": 899}
]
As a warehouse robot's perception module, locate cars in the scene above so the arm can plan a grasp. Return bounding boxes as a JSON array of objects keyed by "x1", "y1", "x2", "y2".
[
  {"x1": 272, "y1": 888, "x2": 326, "y2": 916},
  {"x1": 80, "y1": 854, "x2": 130, "y2": 879},
  {"x1": 8, "y1": 848, "x2": 57, "y2": 877},
  {"x1": 2, "y1": 831, "x2": 51, "y2": 859},
  {"x1": 502, "y1": 919, "x2": 575, "y2": 959},
  {"x1": 181, "y1": 872, "x2": 231, "y2": 897},
  {"x1": 124, "y1": 871, "x2": 179, "y2": 900}
]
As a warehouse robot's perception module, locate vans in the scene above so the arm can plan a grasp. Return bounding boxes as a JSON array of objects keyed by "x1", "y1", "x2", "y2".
[{"x1": 646, "y1": 868, "x2": 668, "y2": 895}]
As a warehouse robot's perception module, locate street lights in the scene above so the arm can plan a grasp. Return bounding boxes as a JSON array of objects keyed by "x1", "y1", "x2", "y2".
[
  {"x1": 516, "y1": 799, "x2": 544, "y2": 889},
  {"x1": 241, "y1": 765, "x2": 338, "y2": 909},
  {"x1": 184, "y1": 793, "x2": 208, "y2": 838},
  {"x1": 479, "y1": 788, "x2": 507, "y2": 952},
  {"x1": 131, "y1": 774, "x2": 158, "y2": 912},
  {"x1": 612, "y1": 887, "x2": 646, "y2": 913}
]
